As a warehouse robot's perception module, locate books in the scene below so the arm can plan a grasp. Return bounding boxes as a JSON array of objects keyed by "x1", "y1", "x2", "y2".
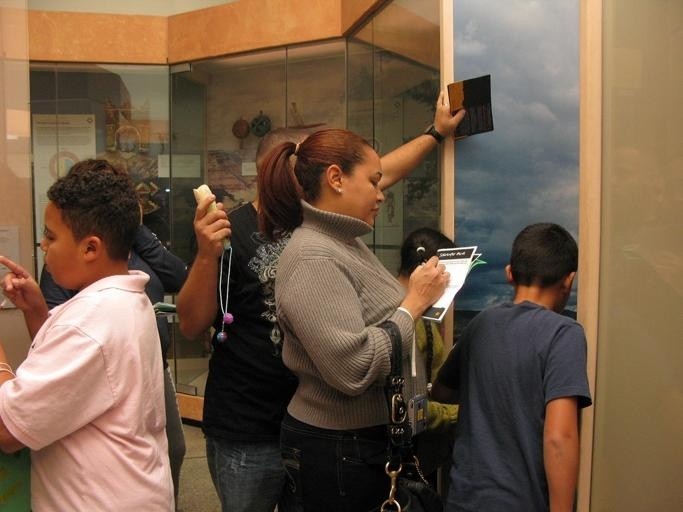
[
  {"x1": 418, "y1": 245, "x2": 487, "y2": 324},
  {"x1": 446, "y1": 73, "x2": 494, "y2": 139},
  {"x1": 152, "y1": 300, "x2": 178, "y2": 317}
]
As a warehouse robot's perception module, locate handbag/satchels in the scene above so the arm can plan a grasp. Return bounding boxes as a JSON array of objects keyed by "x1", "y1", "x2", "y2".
[{"x1": 372, "y1": 319, "x2": 444, "y2": 512}]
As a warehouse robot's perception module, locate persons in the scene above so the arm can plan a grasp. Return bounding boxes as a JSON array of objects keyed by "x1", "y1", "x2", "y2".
[
  {"x1": 35, "y1": 156, "x2": 190, "y2": 512},
  {"x1": 180, "y1": 81, "x2": 468, "y2": 512},
  {"x1": 0, "y1": 168, "x2": 178, "y2": 512},
  {"x1": 252, "y1": 126, "x2": 451, "y2": 512},
  {"x1": 430, "y1": 218, "x2": 595, "y2": 512},
  {"x1": 395, "y1": 218, "x2": 462, "y2": 512}
]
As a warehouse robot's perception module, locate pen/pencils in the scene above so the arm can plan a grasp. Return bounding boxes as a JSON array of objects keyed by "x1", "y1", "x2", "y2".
[{"x1": 417, "y1": 252, "x2": 425, "y2": 263}]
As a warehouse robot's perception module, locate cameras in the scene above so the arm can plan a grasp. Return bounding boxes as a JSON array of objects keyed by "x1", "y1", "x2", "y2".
[{"x1": 406, "y1": 392, "x2": 429, "y2": 436}]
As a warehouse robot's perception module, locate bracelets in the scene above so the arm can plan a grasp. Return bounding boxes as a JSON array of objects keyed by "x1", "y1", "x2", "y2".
[
  {"x1": 0, "y1": 362, "x2": 12, "y2": 371},
  {"x1": 423, "y1": 122, "x2": 446, "y2": 143},
  {"x1": 0, "y1": 369, "x2": 15, "y2": 377}
]
